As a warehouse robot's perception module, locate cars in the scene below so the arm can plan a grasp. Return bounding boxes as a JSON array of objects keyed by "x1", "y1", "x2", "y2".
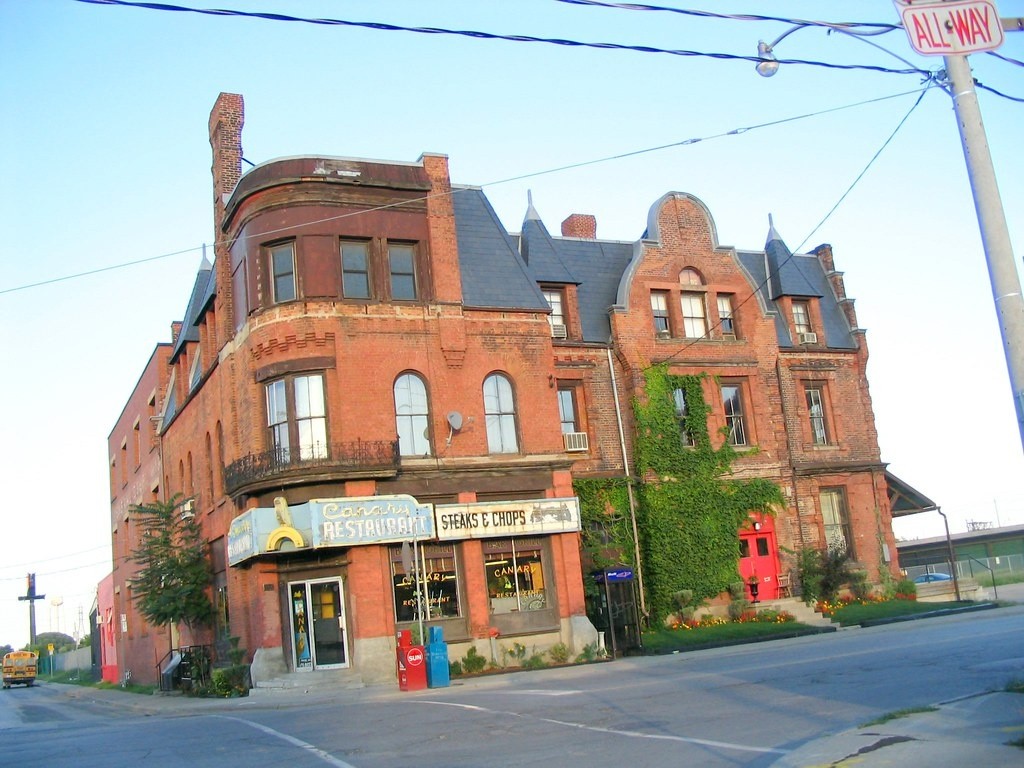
[{"x1": 912, "y1": 573, "x2": 958, "y2": 583}]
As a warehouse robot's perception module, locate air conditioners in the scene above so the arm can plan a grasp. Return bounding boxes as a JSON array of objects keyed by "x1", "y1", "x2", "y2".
[
  {"x1": 96, "y1": 615, "x2": 103, "y2": 625},
  {"x1": 180, "y1": 500, "x2": 195, "y2": 520},
  {"x1": 799, "y1": 333, "x2": 817, "y2": 344},
  {"x1": 564, "y1": 432, "x2": 588, "y2": 452},
  {"x1": 551, "y1": 324, "x2": 567, "y2": 338},
  {"x1": 723, "y1": 335, "x2": 737, "y2": 340}
]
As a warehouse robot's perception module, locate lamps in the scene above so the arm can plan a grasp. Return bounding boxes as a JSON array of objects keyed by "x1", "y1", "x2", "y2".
[{"x1": 754, "y1": 521, "x2": 761, "y2": 530}]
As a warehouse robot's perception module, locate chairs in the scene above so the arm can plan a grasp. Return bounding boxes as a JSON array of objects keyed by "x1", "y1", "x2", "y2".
[{"x1": 776, "y1": 572, "x2": 792, "y2": 599}]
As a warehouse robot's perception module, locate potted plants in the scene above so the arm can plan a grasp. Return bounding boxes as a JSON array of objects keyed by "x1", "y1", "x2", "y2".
[{"x1": 747, "y1": 576, "x2": 760, "y2": 604}]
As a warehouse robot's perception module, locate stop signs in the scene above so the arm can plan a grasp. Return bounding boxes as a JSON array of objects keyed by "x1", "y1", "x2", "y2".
[{"x1": 406, "y1": 649, "x2": 424, "y2": 666}]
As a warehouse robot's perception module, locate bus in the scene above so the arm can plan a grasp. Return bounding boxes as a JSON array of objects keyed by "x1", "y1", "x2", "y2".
[{"x1": 2, "y1": 651, "x2": 36, "y2": 690}]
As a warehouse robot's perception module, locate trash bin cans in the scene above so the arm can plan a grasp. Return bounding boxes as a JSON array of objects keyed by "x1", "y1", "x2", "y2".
[{"x1": 101, "y1": 664, "x2": 119, "y2": 684}]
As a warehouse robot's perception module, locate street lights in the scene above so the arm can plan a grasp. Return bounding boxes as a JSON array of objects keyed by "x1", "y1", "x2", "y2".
[{"x1": 754, "y1": 15, "x2": 1024, "y2": 453}]
